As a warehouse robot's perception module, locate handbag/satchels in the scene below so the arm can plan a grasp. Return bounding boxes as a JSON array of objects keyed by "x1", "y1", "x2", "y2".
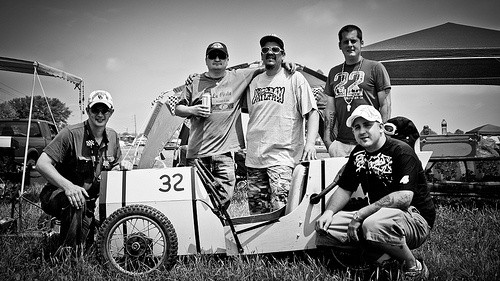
[{"x1": 38, "y1": 183, "x2": 72, "y2": 221}]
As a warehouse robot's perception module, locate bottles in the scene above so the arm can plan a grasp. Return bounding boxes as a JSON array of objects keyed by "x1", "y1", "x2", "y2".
[{"x1": 441, "y1": 119, "x2": 448, "y2": 135}]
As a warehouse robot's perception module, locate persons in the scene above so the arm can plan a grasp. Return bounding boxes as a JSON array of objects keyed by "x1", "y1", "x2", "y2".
[
  {"x1": 35, "y1": 90, "x2": 133, "y2": 269},
  {"x1": 315, "y1": 105, "x2": 436, "y2": 281},
  {"x1": 174, "y1": 42, "x2": 297, "y2": 217},
  {"x1": 324, "y1": 24, "x2": 391, "y2": 157},
  {"x1": 184, "y1": 33, "x2": 321, "y2": 214}
]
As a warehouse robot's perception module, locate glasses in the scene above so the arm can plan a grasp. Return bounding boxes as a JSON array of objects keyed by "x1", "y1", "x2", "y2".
[
  {"x1": 208, "y1": 51, "x2": 226, "y2": 60},
  {"x1": 89, "y1": 107, "x2": 111, "y2": 115},
  {"x1": 261, "y1": 46, "x2": 284, "y2": 55}
]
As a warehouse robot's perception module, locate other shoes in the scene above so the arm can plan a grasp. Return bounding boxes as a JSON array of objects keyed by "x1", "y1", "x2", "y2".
[
  {"x1": 379, "y1": 258, "x2": 400, "y2": 281},
  {"x1": 401, "y1": 259, "x2": 430, "y2": 281}
]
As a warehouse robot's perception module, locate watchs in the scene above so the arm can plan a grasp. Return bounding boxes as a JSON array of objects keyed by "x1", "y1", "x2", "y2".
[{"x1": 353, "y1": 212, "x2": 362, "y2": 223}]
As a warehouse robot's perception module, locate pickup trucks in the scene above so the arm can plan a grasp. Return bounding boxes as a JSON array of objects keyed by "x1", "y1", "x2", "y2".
[{"x1": 0, "y1": 117, "x2": 62, "y2": 184}]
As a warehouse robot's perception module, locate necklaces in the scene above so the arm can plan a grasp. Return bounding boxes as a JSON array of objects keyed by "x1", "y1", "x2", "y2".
[
  {"x1": 85, "y1": 120, "x2": 109, "y2": 182},
  {"x1": 208, "y1": 73, "x2": 225, "y2": 86},
  {"x1": 341, "y1": 56, "x2": 364, "y2": 111}
]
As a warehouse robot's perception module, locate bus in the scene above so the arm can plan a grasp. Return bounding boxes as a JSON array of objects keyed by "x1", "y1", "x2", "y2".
[{"x1": 119, "y1": 135, "x2": 136, "y2": 146}]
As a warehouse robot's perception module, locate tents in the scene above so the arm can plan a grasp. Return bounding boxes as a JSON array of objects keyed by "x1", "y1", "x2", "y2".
[
  {"x1": 0, "y1": 55, "x2": 85, "y2": 194},
  {"x1": 122, "y1": 61, "x2": 327, "y2": 169},
  {"x1": 361, "y1": 22, "x2": 500, "y2": 85}
]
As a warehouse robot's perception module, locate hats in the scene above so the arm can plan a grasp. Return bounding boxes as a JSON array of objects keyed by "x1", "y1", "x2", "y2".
[
  {"x1": 259, "y1": 33, "x2": 285, "y2": 51},
  {"x1": 86, "y1": 90, "x2": 115, "y2": 109},
  {"x1": 346, "y1": 104, "x2": 384, "y2": 128},
  {"x1": 205, "y1": 42, "x2": 228, "y2": 55}
]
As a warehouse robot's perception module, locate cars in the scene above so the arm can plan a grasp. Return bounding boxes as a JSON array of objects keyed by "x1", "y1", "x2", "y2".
[
  {"x1": 164, "y1": 141, "x2": 180, "y2": 150},
  {"x1": 419, "y1": 132, "x2": 500, "y2": 211},
  {"x1": 136, "y1": 138, "x2": 147, "y2": 146}
]
